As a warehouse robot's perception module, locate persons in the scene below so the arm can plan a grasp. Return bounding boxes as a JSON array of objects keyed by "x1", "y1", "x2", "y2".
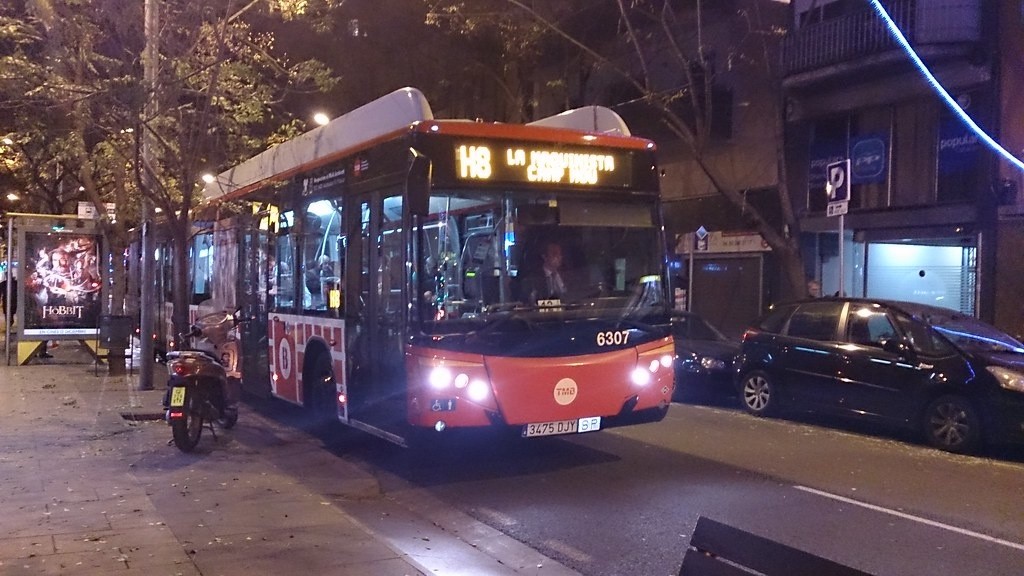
[
  {"x1": 520, "y1": 242, "x2": 596, "y2": 307},
  {"x1": 30, "y1": 236, "x2": 102, "y2": 305},
  {"x1": 255, "y1": 250, "x2": 290, "y2": 305},
  {"x1": 420, "y1": 290, "x2": 436, "y2": 322},
  {"x1": 412, "y1": 253, "x2": 444, "y2": 300},
  {"x1": 307, "y1": 253, "x2": 335, "y2": 310},
  {"x1": 0, "y1": 269, "x2": 18, "y2": 328},
  {"x1": 798, "y1": 279, "x2": 821, "y2": 299}
]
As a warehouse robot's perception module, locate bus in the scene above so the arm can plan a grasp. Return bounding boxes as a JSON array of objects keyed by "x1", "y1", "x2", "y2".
[{"x1": 133, "y1": 84, "x2": 682, "y2": 451}]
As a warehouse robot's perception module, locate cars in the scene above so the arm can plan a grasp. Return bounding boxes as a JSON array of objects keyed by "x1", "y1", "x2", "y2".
[
  {"x1": 732, "y1": 294, "x2": 1024, "y2": 457},
  {"x1": 634, "y1": 308, "x2": 742, "y2": 403}
]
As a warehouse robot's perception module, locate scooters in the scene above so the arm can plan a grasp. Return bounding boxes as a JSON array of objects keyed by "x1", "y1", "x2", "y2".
[{"x1": 163, "y1": 303, "x2": 251, "y2": 454}]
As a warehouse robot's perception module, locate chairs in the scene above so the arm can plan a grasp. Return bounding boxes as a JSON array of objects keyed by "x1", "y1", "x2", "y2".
[
  {"x1": 95, "y1": 314, "x2": 135, "y2": 377},
  {"x1": 460, "y1": 268, "x2": 513, "y2": 302}
]
